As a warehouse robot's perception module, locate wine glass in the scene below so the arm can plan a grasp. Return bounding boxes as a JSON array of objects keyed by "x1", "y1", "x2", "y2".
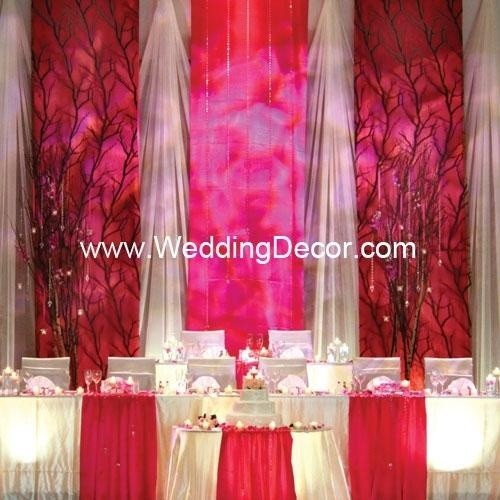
[
  {"x1": 430, "y1": 369, "x2": 439, "y2": 396},
  {"x1": 255, "y1": 333, "x2": 264, "y2": 360},
  {"x1": 354, "y1": 368, "x2": 366, "y2": 395},
  {"x1": 439, "y1": 370, "x2": 447, "y2": 396},
  {"x1": 84, "y1": 369, "x2": 92, "y2": 393},
  {"x1": 23, "y1": 369, "x2": 33, "y2": 393},
  {"x1": 92, "y1": 370, "x2": 102, "y2": 394}
]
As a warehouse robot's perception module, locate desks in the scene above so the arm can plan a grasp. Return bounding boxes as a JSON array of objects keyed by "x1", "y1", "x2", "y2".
[
  {"x1": 166, "y1": 425, "x2": 351, "y2": 500},
  {"x1": 0, "y1": 391, "x2": 500, "y2": 500}
]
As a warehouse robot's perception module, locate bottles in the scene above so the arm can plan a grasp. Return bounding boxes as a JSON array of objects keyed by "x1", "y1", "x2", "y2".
[
  {"x1": 492, "y1": 366, "x2": 500, "y2": 398},
  {"x1": 485, "y1": 374, "x2": 497, "y2": 397}
]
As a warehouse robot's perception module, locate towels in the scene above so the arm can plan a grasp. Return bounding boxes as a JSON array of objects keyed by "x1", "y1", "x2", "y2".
[
  {"x1": 280, "y1": 347, "x2": 304, "y2": 358},
  {"x1": 447, "y1": 377, "x2": 475, "y2": 394},
  {"x1": 202, "y1": 345, "x2": 229, "y2": 356},
  {"x1": 277, "y1": 374, "x2": 307, "y2": 393},
  {"x1": 26, "y1": 375, "x2": 55, "y2": 395},
  {"x1": 192, "y1": 376, "x2": 219, "y2": 390},
  {"x1": 367, "y1": 375, "x2": 392, "y2": 390},
  {"x1": 102, "y1": 377, "x2": 128, "y2": 394}
]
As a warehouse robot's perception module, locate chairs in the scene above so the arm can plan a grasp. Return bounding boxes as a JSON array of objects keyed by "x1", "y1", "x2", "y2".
[
  {"x1": 423, "y1": 357, "x2": 474, "y2": 391},
  {"x1": 258, "y1": 357, "x2": 309, "y2": 387},
  {"x1": 268, "y1": 329, "x2": 313, "y2": 361},
  {"x1": 21, "y1": 356, "x2": 71, "y2": 389},
  {"x1": 182, "y1": 330, "x2": 225, "y2": 354},
  {"x1": 187, "y1": 357, "x2": 236, "y2": 390},
  {"x1": 107, "y1": 357, "x2": 155, "y2": 391},
  {"x1": 353, "y1": 357, "x2": 401, "y2": 390}
]
